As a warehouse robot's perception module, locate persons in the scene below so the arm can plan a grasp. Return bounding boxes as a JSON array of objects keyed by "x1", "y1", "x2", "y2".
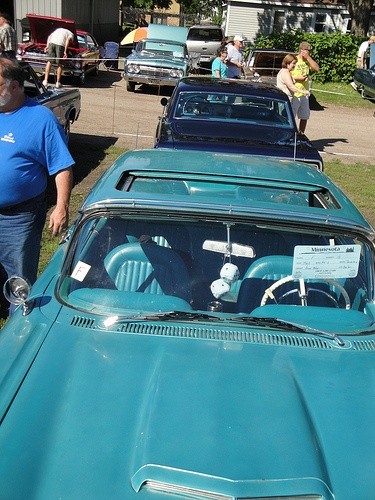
[
  {"x1": 277, "y1": 54, "x2": 310, "y2": 117},
  {"x1": 288, "y1": 42, "x2": 319, "y2": 132},
  {"x1": 207, "y1": 46, "x2": 228, "y2": 102},
  {"x1": 41, "y1": 27, "x2": 74, "y2": 89},
  {"x1": 0, "y1": 16, "x2": 17, "y2": 59},
  {"x1": 351, "y1": 34, "x2": 375, "y2": 90},
  {"x1": 223, "y1": 35, "x2": 244, "y2": 103},
  {"x1": 0, "y1": 55, "x2": 75, "y2": 319}
]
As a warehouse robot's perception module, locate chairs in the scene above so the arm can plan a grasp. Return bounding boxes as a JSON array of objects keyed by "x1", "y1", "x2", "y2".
[
  {"x1": 97, "y1": 242, "x2": 191, "y2": 303},
  {"x1": 236, "y1": 255, "x2": 336, "y2": 312},
  {"x1": 100, "y1": 41, "x2": 121, "y2": 74}
]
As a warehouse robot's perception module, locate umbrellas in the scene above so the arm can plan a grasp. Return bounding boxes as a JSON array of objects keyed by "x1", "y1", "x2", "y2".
[{"x1": 121, "y1": 26, "x2": 148, "y2": 45}]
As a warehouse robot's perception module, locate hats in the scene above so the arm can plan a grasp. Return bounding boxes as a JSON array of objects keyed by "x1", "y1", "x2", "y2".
[{"x1": 234, "y1": 35, "x2": 245, "y2": 42}]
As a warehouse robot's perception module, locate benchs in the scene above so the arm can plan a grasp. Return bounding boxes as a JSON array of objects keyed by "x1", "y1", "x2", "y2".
[{"x1": 103, "y1": 221, "x2": 304, "y2": 304}]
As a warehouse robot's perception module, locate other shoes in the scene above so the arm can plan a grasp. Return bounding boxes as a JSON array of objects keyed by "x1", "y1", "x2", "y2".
[
  {"x1": 351, "y1": 82, "x2": 358, "y2": 91},
  {"x1": 55, "y1": 83, "x2": 62, "y2": 89},
  {"x1": 42, "y1": 81, "x2": 48, "y2": 88}
]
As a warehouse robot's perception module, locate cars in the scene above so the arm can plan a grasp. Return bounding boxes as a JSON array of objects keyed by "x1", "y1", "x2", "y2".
[
  {"x1": 243, "y1": 48, "x2": 313, "y2": 93},
  {"x1": 122, "y1": 23, "x2": 201, "y2": 92},
  {"x1": 0, "y1": 146, "x2": 375, "y2": 499},
  {"x1": 351, "y1": 36, "x2": 375, "y2": 103},
  {"x1": 14, "y1": 13, "x2": 102, "y2": 86},
  {"x1": 153, "y1": 75, "x2": 325, "y2": 173}
]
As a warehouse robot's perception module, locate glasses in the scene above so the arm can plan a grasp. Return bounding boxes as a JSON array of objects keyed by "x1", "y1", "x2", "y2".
[{"x1": 223, "y1": 51, "x2": 228, "y2": 55}]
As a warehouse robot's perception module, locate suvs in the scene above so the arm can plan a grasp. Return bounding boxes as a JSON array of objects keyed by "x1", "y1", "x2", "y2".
[{"x1": 186, "y1": 25, "x2": 229, "y2": 62}]
